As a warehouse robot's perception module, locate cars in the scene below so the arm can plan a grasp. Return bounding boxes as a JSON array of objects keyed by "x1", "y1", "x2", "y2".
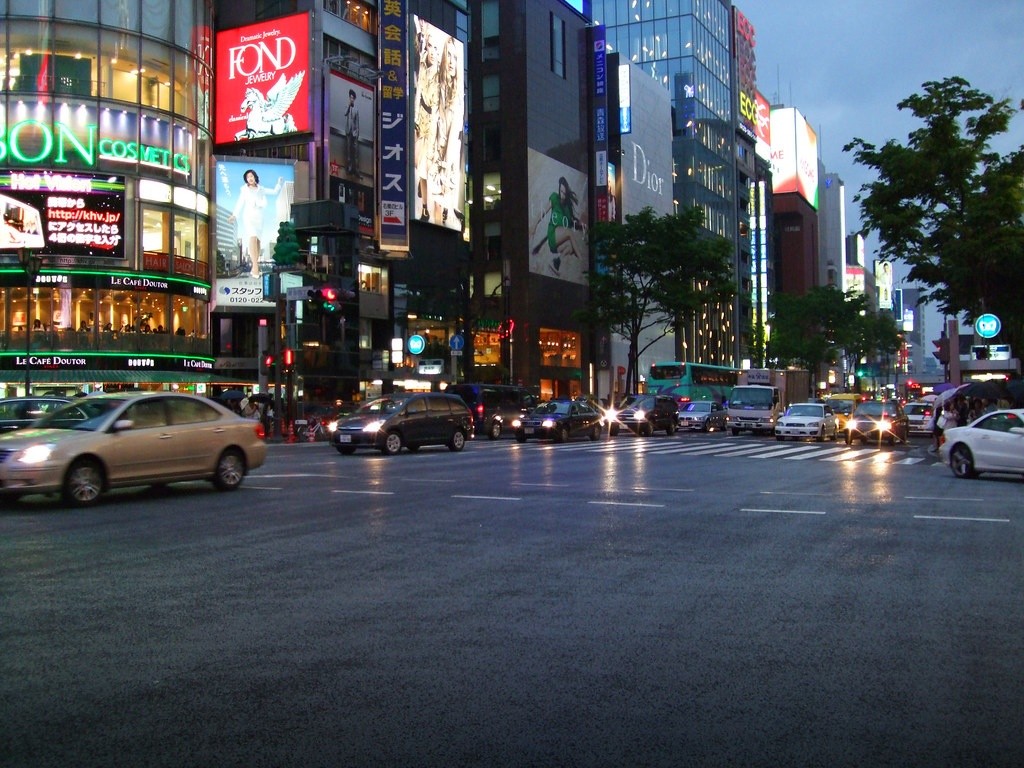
[
  {"x1": 677, "y1": 400, "x2": 728, "y2": 433},
  {"x1": 776, "y1": 401, "x2": 840, "y2": 443},
  {"x1": 0, "y1": 396, "x2": 103, "y2": 433},
  {"x1": 840, "y1": 399, "x2": 910, "y2": 447},
  {"x1": 902, "y1": 401, "x2": 935, "y2": 438},
  {"x1": 516, "y1": 401, "x2": 605, "y2": 443},
  {"x1": 1, "y1": 387, "x2": 267, "y2": 510},
  {"x1": 938, "y1": 408, "x2": 1024, "y2": 478}
]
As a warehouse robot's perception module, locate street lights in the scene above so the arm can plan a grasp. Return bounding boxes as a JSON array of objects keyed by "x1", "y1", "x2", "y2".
[{"x1": 15, "y1": 245, "x2": 43, "y2": 398}]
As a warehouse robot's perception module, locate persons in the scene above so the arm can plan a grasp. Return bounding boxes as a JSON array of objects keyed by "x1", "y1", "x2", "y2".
[
  {"x1": 529, "y1": 176, "x2": 588, "y2": 276},
  {"x1": 18, "y1": 319, "x2": 198, "y2": 346},
  {"x1": 228, "y1": 398, "x2": 288, "y2": 436},
  {"x1": 876, "y1": 263, "x2": 892, "y2": 286},
  {"x1": 344, "y1": 89, "x2": 362, "y2": 178},
  {"x1": 228, "y1": 169, "x2": 283, "y2": 278},
  {"x1": 929, "y1": 397, "x2": 1024, "y2": 453},
  {"x1": 414, "y1": 15, "x2": 462, "y2": 231}
]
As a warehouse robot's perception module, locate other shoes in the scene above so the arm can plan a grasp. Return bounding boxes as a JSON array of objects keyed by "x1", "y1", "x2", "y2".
[
  {"x1": 927, "y1": 443, "x2": 938, "y2": 452},
  {"x1": 354, "y1": 163, "x2": 364, "y2": 180},
  {"x1": 249, "y1": 267, "x2": 261, "y2": 280},
  {"x1": 550, "y1": 256, "x2": 564, "y2": 275}
]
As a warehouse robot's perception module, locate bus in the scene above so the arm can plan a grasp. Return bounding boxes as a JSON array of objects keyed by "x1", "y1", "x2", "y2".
[{"x1": 646, "y1": 361, "x2": 741, "y2": 413}]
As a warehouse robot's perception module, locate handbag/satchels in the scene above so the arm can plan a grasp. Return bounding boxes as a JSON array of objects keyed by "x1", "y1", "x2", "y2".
[{"x1": 937, "y1": 409, "x2": 959, "y2": 428}]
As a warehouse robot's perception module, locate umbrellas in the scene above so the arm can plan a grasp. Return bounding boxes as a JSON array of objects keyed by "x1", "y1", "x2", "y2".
[
  {"x1": 218, "y1": 390, "x2": 273, "y2": 410},
  {"x1": 930, "y1": 378, "x2": 1024, "y2": 414}
]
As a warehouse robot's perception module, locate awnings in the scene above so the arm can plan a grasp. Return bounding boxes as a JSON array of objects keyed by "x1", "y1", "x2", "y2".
[
  {"x1": 474, "y1": 366, "x2": 509, "y2": 382},
  {"x1": 538, "y1": 366, "x2": 582, "y2": 380},
  {"x1": 0, "y1": 370, "x2": 255, "y2": 383}
]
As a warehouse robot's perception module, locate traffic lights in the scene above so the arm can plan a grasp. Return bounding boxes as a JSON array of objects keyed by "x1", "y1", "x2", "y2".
[
  {"x1": 907, "y1": 378, "x2": 913, "y2": 387},
  {"x1": 932, "y1": 337, "x2": 950, "y2": 365},
  {"x1": 264, "y1": 352, "x2": 276, "y2": 369},
  {"x1": 284, "y1": 347, "x2": 293, "y2": 367},
  {"x1": 307, "y1": 285, "x2": 340, "y2": 311},
  {"x1": 856, "y1": 364, "x2": 864, "y2": 377}
]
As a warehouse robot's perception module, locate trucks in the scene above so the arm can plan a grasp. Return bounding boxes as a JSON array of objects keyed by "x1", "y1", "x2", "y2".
[{"x1": 722, "y1": 368, "x2": 810, "y2": 438}]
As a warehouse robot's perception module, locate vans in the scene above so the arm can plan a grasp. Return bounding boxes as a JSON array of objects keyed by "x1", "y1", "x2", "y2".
[
  {"x1": 446, "y1": 383, "x2": 543, "y2": 441},
  {"x1": 819, "y1": 393, "x2": 862, "y2": 438}
]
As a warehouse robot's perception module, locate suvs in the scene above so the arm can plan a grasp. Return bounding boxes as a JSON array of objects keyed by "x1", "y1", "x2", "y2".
[
  {"x1": 329, "y1": 391, "x2": 475, "y2": 457},
  {"x1": 605, "y1": 393, "x2": 680, "y2": 437}
]
